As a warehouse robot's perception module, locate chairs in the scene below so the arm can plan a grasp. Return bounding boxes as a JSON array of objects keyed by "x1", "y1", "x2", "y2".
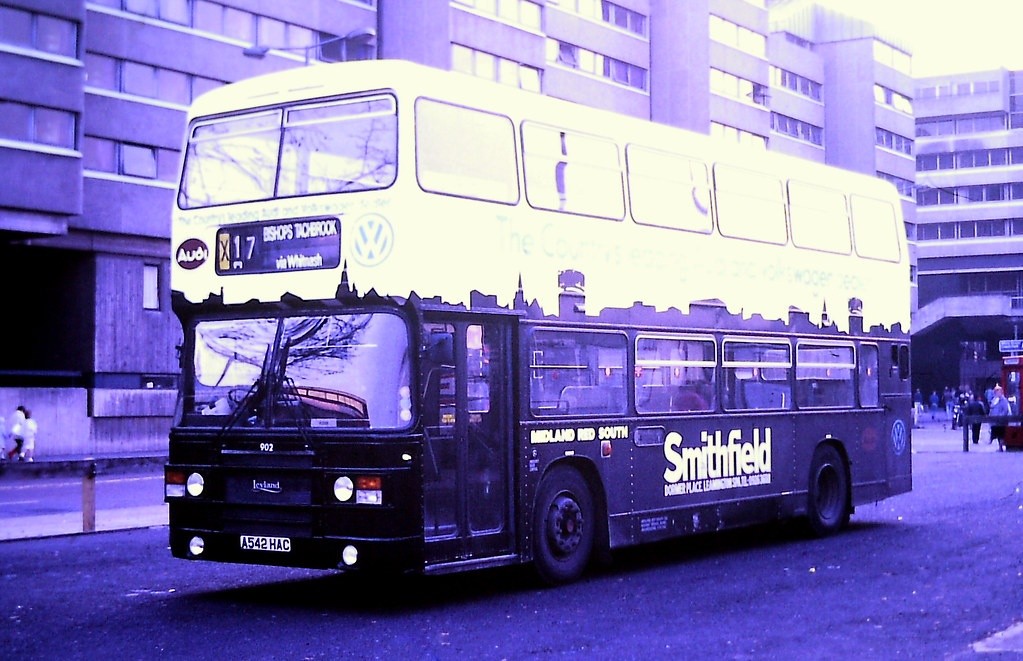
[{"x1": 423, "y1": 353, "x2": 879, "y2": 442}]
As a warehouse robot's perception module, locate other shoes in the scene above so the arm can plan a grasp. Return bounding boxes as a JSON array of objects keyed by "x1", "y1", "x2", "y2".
[
  {"x1": 8, "y1": 449, "x2": 17, "y2": 461},
  {"x1": 19, "y1": 452, "x2": 26, "y2": 460},
  {"x1": 27, "y1": 457, "x2": 33, "y2": 462},
  {"x1": 972, "y1": 438, "x2": 979, "y2": 445}
]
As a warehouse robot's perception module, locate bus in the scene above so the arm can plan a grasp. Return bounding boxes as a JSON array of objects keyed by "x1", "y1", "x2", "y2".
[{"x1": 158, "y1": 57, "x2": 914, "y2": 583}]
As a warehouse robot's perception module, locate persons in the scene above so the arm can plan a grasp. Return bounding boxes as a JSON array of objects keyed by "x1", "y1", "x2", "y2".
[
  {"x1": 19, "y1": 409, "x2": 37, "y2": 464},
  {"x1": 672, "y1": 380, "x2": 709, "y2": 413},
  {"x1": 911, "y1": 383, "x2": 996, "y2": 422},
  {"x1": 7, "y1": 405, "x2": 24, "y2": 459},
  {"x1": 1, "y1": 417, "x2": 13, "y2": 458},
  {"x1": 967, "y1": 392, "x2": 988, "y2": 442},
  {"x1": 987, "y1": 387, "x2": 1012, "y2": 453}
]
